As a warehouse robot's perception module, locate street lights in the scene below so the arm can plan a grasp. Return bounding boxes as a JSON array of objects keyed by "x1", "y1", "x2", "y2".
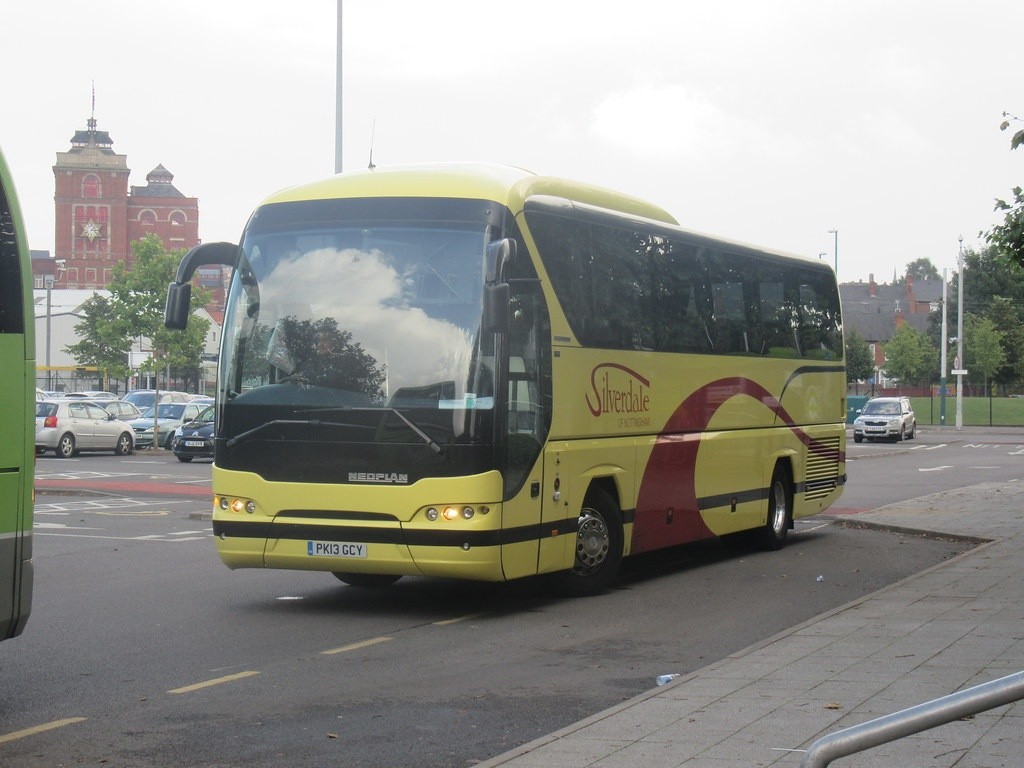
[
  {"x1": 955, "y1": 234, "x2": 965, "y2": 431},
  {"x1": 45, "y1": 258, "x2": 67, "y2": 391},
  {"x1": 825, "y1": 227, "x2": 839, "y2": 278}
]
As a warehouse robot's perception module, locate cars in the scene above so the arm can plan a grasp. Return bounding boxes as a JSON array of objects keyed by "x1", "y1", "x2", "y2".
[
  {"x1": 35, "y1": 400, "x2": 136, "y2": 459},
  {"x1": 128, "y1": 402, "x2": 214, "y2": 451},
  {"x1": 171, "y1": 402, "x2": 216, "y2": 463},
  {"x1": 35, "y1": 388, "x2": 216, "y2": 423}
]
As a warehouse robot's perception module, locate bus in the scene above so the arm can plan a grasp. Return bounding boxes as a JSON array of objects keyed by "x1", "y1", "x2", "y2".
[
  {"x1": 0, "y1": 146, "x2": 34, "y2": 641},
  {"x1": 161, "y1": 161, "x2": 849, "y2": 596}
]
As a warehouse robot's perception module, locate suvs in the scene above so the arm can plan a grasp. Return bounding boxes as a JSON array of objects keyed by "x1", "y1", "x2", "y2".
[{"x1": 852, "y1": 396, "x2": 916, "y2": 444}]
